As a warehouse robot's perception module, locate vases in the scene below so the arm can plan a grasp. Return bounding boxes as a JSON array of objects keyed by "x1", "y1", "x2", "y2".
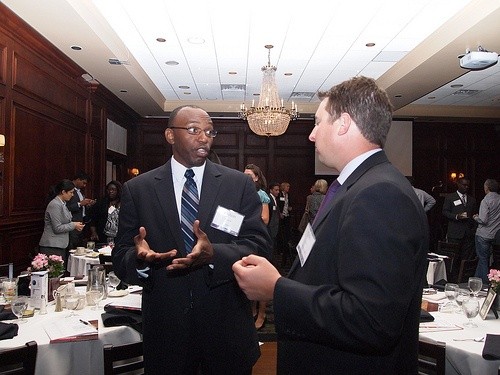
[{"x1": 48, "y1": 279, "x2": 60, "y2": 302}]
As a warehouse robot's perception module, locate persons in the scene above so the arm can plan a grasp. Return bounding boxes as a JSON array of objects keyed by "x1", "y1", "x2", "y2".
[
  {"x1": 270, "y1": 183, "x2": 279, "y2": 238},
  {"x1": 279, "y1": 181, "x2": 292, "y2": 251},
  {"x1": 232, "y1": 76, "x2": 429, "y2": 375},
  {"x1": 67, "y1": 171, "x2": 97, "y2": 247},
  {"x1": 298, "y1": 179, "x2": 328, "y2": 233},
  {"x1": 243, "y1": 164, "x2": 269, "y2": 330},
  {"x1": 88, "y1": 181, "x2": 122, "y2": 244},
  {"x1": 38, "y1": 179, "x2": 86, "y2": 261},
  {"x1": 406, "y1": 175, "x2": 436, "y2": 214},
  {"x1": 441, "y1": 178, "x2": 478, "y2": 281},
  {"x1": 473, "y1": 179, "x2": 500, "y2": 281},
  {"x1": 111, "y1": 105, "x2": 273, "y2": 375}
]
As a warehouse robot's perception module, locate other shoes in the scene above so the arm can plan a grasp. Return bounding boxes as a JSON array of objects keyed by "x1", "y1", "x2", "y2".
[{"x1": 255, "y1": 313, "x2": 266, "y2": 329}]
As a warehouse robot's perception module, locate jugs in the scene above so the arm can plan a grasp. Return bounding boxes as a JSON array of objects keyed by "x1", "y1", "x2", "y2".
[{"x1": 86, "y1": 263, "x2": 107, "y2": 300}]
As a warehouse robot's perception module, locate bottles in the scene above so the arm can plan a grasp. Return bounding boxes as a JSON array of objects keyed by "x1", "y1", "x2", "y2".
[
  {"x1": 55, "y1": 292, "x2": 62, "y2": 312},
  {"x1": 40, "y1": 294, "x2": 46, "y2": 315}
]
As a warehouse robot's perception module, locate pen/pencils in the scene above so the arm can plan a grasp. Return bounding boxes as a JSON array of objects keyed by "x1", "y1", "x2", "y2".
[{"x1": 79, "y1": 320, "x2": 98, "y2": 330}]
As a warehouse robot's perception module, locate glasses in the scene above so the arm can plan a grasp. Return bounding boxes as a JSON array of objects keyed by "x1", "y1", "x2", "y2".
[{"x1": 172, "y1": 126, "x2": 217, "y2": 137}]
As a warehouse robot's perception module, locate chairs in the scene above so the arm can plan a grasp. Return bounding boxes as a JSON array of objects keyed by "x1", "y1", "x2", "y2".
[
  {"x1": 0, "y1": 341, "x2": 38, "y2": 375},
  {"x1": 0, "y1": 263, "x2": 14, "y2": 281},
  {"x1": 103, "y1": 342, "x2": 143, "y2": 375}
]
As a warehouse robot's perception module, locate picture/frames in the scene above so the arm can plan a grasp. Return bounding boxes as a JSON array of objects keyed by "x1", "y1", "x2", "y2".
[{"x1": 479, "y1": 288, "x2": 499, "y2": 320}]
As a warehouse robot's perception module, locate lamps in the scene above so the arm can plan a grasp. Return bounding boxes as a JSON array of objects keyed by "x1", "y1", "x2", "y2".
[
  {"x1": 238, "y1": 48, "x2": 300, "y2": 136},
  {"x1": 0, "y1": 135, "x2": 5, "y2": 162}
]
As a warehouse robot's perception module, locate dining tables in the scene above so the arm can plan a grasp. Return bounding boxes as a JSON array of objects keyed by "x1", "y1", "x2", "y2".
[
  {"x1": 417, "y1": 290, "x2": 500, "y2": 375},
  {"x1": 426, "y1": 252, "x2": 444, "y2": 288},
  {"x1": 68, "y1": 250, "x2": 113, "y2": 280},
  {"x1": 0, "y1": 275, "x2": 143, "y2": 374}
]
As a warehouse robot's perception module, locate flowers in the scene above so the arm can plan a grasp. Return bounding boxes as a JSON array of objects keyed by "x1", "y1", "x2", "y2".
[
  {"x1": 32, "y1": 253, "x2": 64, "y2": 279},
  {"x1": 487, "y1": 269, "x2": 500, "y2": 291}
]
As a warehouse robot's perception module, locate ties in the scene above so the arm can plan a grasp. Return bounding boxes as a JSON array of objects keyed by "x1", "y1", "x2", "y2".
[
  {"x1": 312, "y1": 179, "x2": 342, "y2": 231},
  {"x1": 180, "y1": 168, "x2": 200, "y2": 255},
  {"x1": 462, "y1": 195, "x2": 465, "y2": 205}
]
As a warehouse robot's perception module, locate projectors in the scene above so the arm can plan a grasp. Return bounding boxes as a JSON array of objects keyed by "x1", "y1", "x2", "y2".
[{"x1": 458, "y1": 48, "x2": 498, "y2": 70}]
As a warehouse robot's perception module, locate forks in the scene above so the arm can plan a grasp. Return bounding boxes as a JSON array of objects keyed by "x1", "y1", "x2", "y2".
[{"x1": 453, "y1": 336, "x2": 484, "y2": 342}]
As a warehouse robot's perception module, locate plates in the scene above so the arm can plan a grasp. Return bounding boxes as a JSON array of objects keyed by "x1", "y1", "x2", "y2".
[
  {"x1": 69, "y1": 249, "x2": 93, "y2": 253},
  {"x1": 86, "y1": 251, "x2": 100, "y2": 257}
]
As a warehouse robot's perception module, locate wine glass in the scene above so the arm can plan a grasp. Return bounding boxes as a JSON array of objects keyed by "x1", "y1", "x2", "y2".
[
  {"x1": 11, "y1": 296, "x2": 28, "y2": 324},
  {"x1": 456, "y1": 289, "x2": 470, "y2": 307},
  {"x1": 109, "y1": 272, "x2": 121, "y2": 295},
  {"x1": 468, "y1": 278, "x2": 482, "y2": 297},
  {"x1": 90, "y1": 285, "x2": 104, "y2": 311},
  {"x1": 3, "y1": 279, "x2": 16, "y2": 302},
  {"x1": 107, "y1": 238, "x2": 114, "y2": 250},
  {"x1": 445, "y1": 283, "x2": 459, "y2": 310},
  {"x1": 462, "y1": 298, "x2": 480, "y2": 328}
]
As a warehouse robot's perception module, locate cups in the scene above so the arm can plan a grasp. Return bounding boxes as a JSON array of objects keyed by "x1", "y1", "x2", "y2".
[
  {"x1": 77, "y1": 247, "x2": 85, "y2": 254},
  {"x1": 86, "y1": 291, "x2": 103, "y2": 305},
  {"x1": 66, "y1": 295, "x2": 85, "y2": 311}
]
